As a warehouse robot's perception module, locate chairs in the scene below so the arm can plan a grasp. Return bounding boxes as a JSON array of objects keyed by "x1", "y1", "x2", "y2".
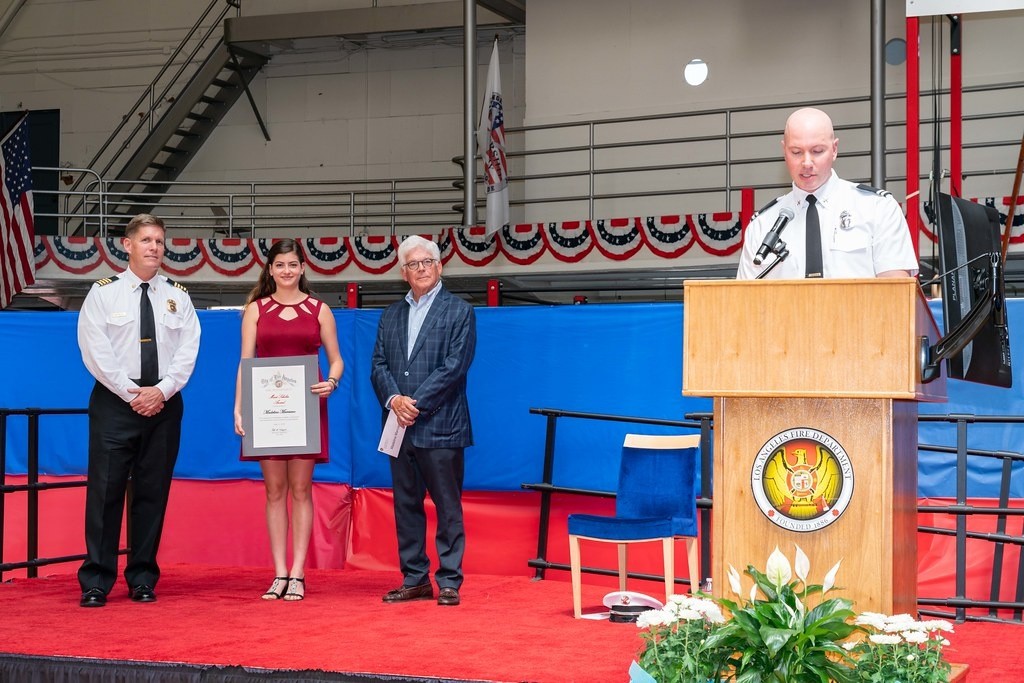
[
  {"x1": 567, "y1": 434, "x2": 701, "y2": 620},
  {"x1": 210, "y1": 202, "x2": 251, "y2": 239}
]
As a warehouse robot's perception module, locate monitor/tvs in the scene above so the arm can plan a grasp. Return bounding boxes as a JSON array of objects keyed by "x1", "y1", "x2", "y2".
[{"x1": 934, "y1": 191, "x2": 1012, "y2": 389}]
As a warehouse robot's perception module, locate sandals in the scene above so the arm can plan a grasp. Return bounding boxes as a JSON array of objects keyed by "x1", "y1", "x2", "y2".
[
  {"x1": 261, "y1": 576, "x2": 289, "y2": 601},
  {"x1": 283, "y1": 577, "x2": 305, "y2": 601}
]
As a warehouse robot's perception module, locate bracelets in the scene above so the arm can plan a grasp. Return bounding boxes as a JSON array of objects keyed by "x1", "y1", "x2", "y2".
[
  {"x1": 327, "y1": 377, "x2": 338, "y2": 390},
  {"x1": 390, "y1": 395, "x2": 400, "y2": 408}
]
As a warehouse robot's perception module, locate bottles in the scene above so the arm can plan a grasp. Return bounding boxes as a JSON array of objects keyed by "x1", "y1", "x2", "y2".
[{"x1": 701, "y1": 577, "x2": 712, "y2": 596}]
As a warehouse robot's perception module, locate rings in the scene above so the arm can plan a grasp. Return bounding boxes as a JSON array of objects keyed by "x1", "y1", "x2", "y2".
[{"x1": 141, "y1": 409, "x2": 145, "y2": 413}]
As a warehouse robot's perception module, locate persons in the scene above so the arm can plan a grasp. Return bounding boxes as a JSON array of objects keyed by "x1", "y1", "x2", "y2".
[
  {"x1": 736, "y1": 107, "x2": 919, "y2": 279},
  {"x1": 234, "y1": 237, "x2": 344, "y2": 601},
  {"x1": 77, "y1": 214, "x2": 201, "y2": 607},
  {"x1": 370, "y1": 234, "x2": 477, "y2": 606}
]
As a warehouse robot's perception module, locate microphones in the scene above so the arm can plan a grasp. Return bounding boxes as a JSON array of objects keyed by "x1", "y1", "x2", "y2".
[{"x1": 754, "y1": 208, "x2": 794, "y2": 265}]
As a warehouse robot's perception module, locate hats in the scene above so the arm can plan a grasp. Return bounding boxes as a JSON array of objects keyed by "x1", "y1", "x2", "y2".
[{"x1": 603, "y1": 591, "x2": 665, "y2": 624}]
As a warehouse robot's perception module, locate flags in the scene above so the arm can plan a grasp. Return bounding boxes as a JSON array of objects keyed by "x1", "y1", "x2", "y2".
[
  {"x1": 0, "y1": 112, "x2": 35, "y2": 310},
  {"x1": 476, "y1": 39, "x2": 510, "y2": 245}
]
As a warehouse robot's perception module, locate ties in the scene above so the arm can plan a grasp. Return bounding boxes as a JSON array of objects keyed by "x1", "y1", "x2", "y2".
[
  {"x1": 140, "y1": 282, "x2": 160, "y2": 389},
  {"x1": 805, "y1": 193, "x2": 825, "y2": 279}
]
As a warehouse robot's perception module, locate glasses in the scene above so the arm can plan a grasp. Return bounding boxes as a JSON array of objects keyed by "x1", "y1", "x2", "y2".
[{"x1": 402, "y1": 257, "x2": 438, "y2": 272}]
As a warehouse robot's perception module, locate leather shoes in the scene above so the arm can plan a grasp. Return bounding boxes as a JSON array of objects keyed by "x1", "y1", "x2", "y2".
[
  {"x1": 437, "y1": 587, "x2": 461, "y2": 606},
  {"x1": 128, "y1": 582, "x2": 157, "y2": 603},
  {"x1": 81, "y1": 587, "x2": 108, "y2": 606},
  {"x1": 382, "y1": 582, "x2": 434, "y2": 602}
]
